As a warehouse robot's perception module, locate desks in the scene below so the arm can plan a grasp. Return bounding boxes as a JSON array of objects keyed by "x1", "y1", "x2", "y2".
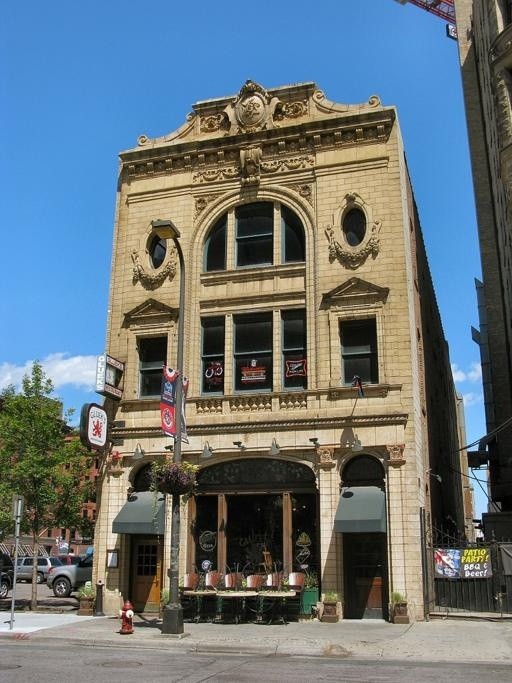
[{"x1": 178, "y1": 562, "x2": 306, "y2": 625}]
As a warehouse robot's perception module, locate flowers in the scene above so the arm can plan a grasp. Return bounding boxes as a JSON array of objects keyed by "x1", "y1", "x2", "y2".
[{"x1": 145, "y1": 461, "x2": 202, "y2": 545}]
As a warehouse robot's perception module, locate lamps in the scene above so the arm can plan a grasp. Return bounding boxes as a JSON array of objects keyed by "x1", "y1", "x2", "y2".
[{"x1": 130, "y1": 434, "x2": 364, "y2": 458}]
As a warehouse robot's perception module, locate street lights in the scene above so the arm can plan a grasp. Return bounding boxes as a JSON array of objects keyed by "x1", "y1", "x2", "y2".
[{"x1": 152, "y1": 220, "x2": 185, "y2": 634}]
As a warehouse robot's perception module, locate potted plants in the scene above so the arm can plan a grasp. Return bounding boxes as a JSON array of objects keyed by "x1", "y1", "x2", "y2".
[
  {"x1": 392, "y1": 592, "x2": 408, "y2": 617},
  {"x1": 322, "y1": 591, "x2": 338, "y2": 616}
]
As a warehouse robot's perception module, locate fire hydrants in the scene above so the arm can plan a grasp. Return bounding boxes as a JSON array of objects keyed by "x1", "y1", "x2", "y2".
[{"x1": 118, "y1": 600, "x2": 135, "y2": 634}]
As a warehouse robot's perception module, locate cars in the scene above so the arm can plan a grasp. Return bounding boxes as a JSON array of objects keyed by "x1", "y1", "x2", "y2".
[{"x1": 0, "y1": 553, "x2": 93, "y2": 599}]
